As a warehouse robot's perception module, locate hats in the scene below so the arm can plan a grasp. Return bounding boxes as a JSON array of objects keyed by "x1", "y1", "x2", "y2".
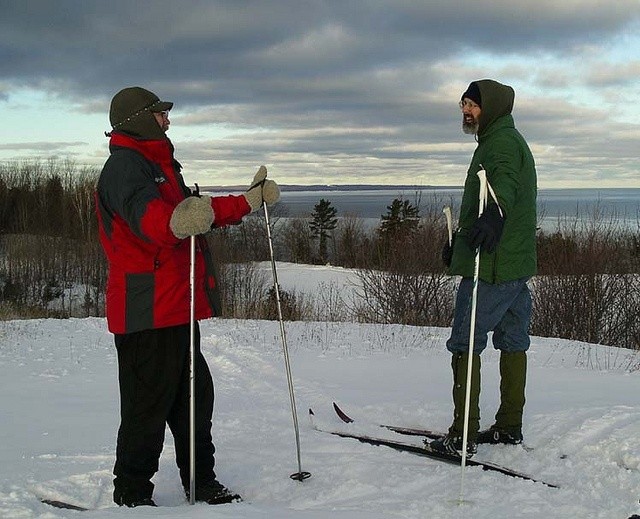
[
  {"x1": 461, "y1": 81, "x2": 482, "y2": 110},
  {"x1": 109, "y1": 86, "x2": 173, "y2": 129}
]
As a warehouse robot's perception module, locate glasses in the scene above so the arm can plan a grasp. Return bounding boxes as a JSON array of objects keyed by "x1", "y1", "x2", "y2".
[
  {"x1": 458, "y1": 100, "x2": 479, "y2": 111},
  {"x1": 153, "y1": 110, "x2": 168, "y2": 120}
]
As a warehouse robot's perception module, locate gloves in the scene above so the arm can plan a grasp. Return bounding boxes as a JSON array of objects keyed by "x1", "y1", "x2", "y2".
[
  {"x1": 170, "y1": 195, "x2": 215, "y2": 240},
  {"x1": 442, "y1": 227, "x2": 460, "y2": 268},
  {"x1": 242, "y1": 165, "x2": 280, "y2": 216},
  {"x1": 462, "y1": 202, "x2": 507, "y2": 255}
]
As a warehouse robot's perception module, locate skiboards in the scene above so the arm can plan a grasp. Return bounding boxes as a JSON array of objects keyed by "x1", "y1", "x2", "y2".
[
  {"x1": 41, "y1": 499, "x2": 89, "y2": 511},
  {"x1": 309, "y1": 402, "x2": 567, "y2": 490}
]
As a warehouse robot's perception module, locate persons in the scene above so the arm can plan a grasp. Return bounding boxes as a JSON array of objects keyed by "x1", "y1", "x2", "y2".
[
  {"x1": 94, "y1": 87, "x2": 280, "y2": 509},
  {"x1": 427, "y1": 79, "x2": 539, "y2": 461}
]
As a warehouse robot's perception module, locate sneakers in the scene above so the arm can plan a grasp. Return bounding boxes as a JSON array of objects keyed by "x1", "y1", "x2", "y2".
[
  {"x1": 111, "y1": 491, "x2": 158, "y2": 509},
  {"x1": 430, "y1": 425, "x2": 478, "y2": 459},
  {"x1": 476, "y1": 424, "x2": 523, "y2": 445},
  {"x1": 185, "y1": 487, "x2": 243, "y2": 505}
]
